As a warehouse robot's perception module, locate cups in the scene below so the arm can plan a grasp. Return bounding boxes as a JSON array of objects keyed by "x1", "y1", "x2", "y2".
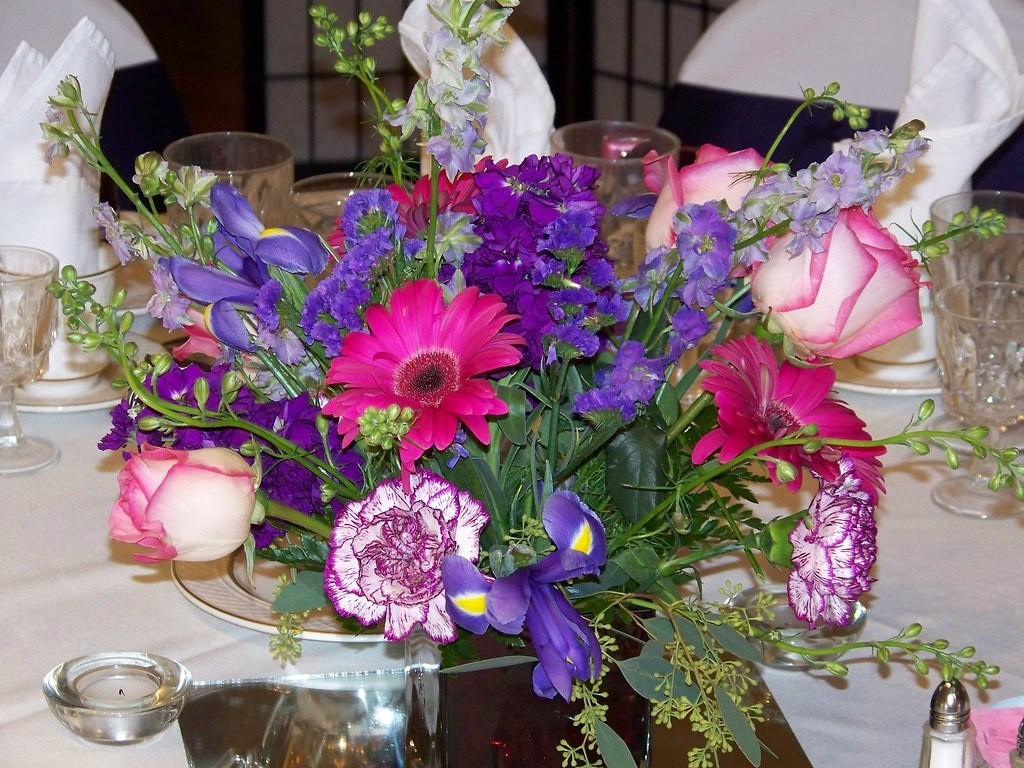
[
  {"x1": 291, "y1": 170, "x2": 417, "y2": 287},
  {"x1": 547, "y1": 119, "x2": 680, "y2": 280},
  {"x1": 161, "y1": 131, "x2": 291, "y2": 232},
  {"x1": 856, "y1": 263, "x2": 941, "y2": 380},
  {"x1": 0, "y1": 235, "x2": 122, "y2": 401},
  {"x1": 928, "y1": 191, "x2": 1024, "y2": 283}
]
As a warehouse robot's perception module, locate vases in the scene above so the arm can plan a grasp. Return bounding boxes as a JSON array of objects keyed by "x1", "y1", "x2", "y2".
[{"x1": 432, "y1": 606, "x2": 650, "y2": 768}]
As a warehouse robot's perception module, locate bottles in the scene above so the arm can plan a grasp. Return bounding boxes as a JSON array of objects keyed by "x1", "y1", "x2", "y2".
[
  {"x1": 1008, "y1": 717, "x2": 1024, "y2": 768},
  {"x1": 916, "y1": 675, "x2": 981, "y2": 768}
]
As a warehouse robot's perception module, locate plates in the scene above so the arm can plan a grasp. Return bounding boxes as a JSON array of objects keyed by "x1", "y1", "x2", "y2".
[
  {"x1": 13, "y1": 330, "x2": 171, "y2": 414},
  {"x1": 822, "y1": 359, "x2": 943, "y2": 397},
  {"x1": 165, "y1": 553, "x2": 405, "y2": 644}
]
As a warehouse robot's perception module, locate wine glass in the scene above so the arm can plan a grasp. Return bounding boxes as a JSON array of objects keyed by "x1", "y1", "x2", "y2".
[
  {"x1": 931, "y1": 284, "x2": 1024, "y2": 522},
  {"x1": 0, "y1": 245, "x2": 60, "y2": 480}
]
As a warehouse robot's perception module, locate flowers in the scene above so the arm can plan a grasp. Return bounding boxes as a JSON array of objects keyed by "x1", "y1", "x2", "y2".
[{"x1": 37, "y1": 0, "x2": 1024, "y2": 768}]
